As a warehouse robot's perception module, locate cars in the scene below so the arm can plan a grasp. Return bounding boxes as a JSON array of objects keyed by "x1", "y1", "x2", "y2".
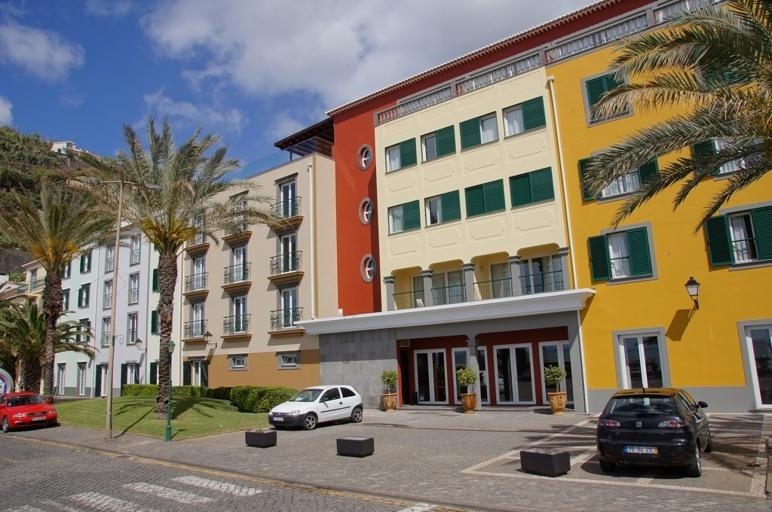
[
  {"x1": 0, "y1": 391, "x2": 57, "y2": 433},
  {"x1": 479, "y1": 370, "x2": 505, "y2": 392},
  {"x1": 753, "y1": 338, "x2": 772, "y2": 375},
  {"x1": 268, "y1": 384, "x2": 363, "y2": 430},
  {"x1": 596, "y1": 388, "x2": 712, "y2": 477},
  {"x1": 626, "y1": 340, "x2": 660, "y2": 376}
]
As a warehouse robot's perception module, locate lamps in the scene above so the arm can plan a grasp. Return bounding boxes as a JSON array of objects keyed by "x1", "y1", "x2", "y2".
[
  {"x1": 203, "y1": 330, "x2": 217, "y2": 349},
  {"x1": 135, "y1": 338, "x2": 144, "y2": 351},
  {"x1": 684, "y1": 276, "x2": 701, "y2": 309}
]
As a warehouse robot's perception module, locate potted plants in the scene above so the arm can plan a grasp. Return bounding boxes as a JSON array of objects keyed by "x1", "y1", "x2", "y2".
[
  {"x1": 544, "y1": 364, "x2": 567, "y2": 415},
  {"x1": 380, "y1": 371, "x2": 398, "y2": 414},
  {"x1": 455, "y1": 368, "x2": 478, "y2": 414}
]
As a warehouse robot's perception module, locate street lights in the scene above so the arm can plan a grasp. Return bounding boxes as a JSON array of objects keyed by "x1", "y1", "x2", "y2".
[
  {"x1": 83, "y1": 176, "x2": 163, "y2": 438},
  {"x1": 165, "y1": 341, "x2": 176, "y2": 441}
]
